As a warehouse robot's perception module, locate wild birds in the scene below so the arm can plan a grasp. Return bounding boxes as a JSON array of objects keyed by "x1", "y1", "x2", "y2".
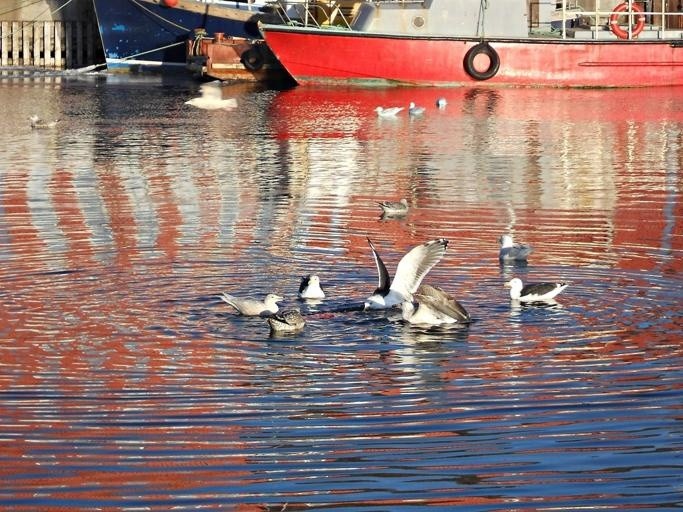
[
  {"x1": 375, "y1": 198, "x2": 412, "y2": 213},
  {"x1": 267, "y1": 310, "x2": 305, "y2": 331},
  {"x1": 298, "y1": 275, "x2": 325, "y2": 299},
  {"x1": 373, "y1": 101, "x2": 427, "y2": 115},
  {"x1": 219, "y1": 292, "x2": 284, "y2": 316},
  {"x1": 183, "y1": 82, "x2": 238, "y2": 110},
  {"x1": 362, "y1": 236, "x2": 472, "y2": 325},
  {"x1": 499, "y1": 235, "x2": 536, "y2": 260},
  {"x1": 436, "y1": 97, "x2": 447, "y2": 107},
  {"x1": 504, "y1": 277, "x2": 569, "y2": 301},
  {"x1": 26, "y1": 114, "x2": 61, "y2": 129}
]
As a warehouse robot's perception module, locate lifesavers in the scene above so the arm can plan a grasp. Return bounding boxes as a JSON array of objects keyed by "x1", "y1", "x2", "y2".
[{"x1": 610, "y1": 2, "x2": 645, "y2": 39}]
